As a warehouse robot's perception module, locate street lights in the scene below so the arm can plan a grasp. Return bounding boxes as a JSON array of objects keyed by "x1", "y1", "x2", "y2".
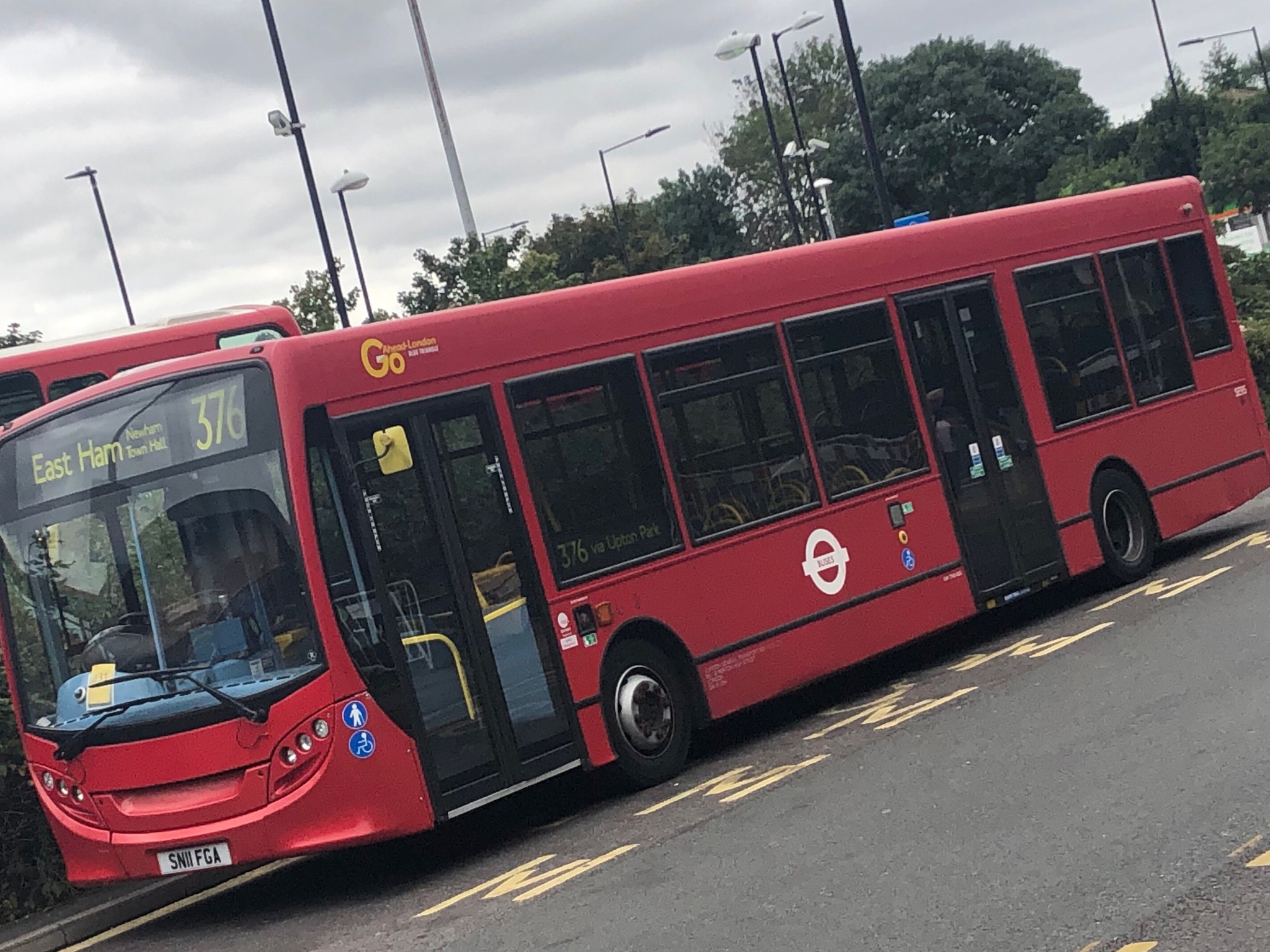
[
  {"x1": 1177, "y1": 26, "x2": 1270, "y2": 95},
  {"x1": 482, "y1": 218, "x2": 529, "y2": 250},
  {"x1": 598, "y1": 123, "x2": 673, "y2": 278},
  {"x1": 770, "y1": 11, "x2": 832, "y2": 241},
  {"x1": 329, "y1": 167, "x2": 379, "y2": 322},
  {"x1": 712, "y1": 27, "x2": 804, "y2": 246},
  {"x1": 66, "y1": 165, "x2": 136, "y2": 327}
]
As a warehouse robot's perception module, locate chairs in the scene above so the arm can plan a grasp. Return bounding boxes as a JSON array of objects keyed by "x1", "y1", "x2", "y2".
[{"x1": 475, "y1": 314, "x2": 1224, "y2": 612}]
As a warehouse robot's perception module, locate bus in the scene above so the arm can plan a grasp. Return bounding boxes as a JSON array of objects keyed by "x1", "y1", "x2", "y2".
[{"x1": 0, "y1": 176, "x2": 1270, "y2": 886}]
